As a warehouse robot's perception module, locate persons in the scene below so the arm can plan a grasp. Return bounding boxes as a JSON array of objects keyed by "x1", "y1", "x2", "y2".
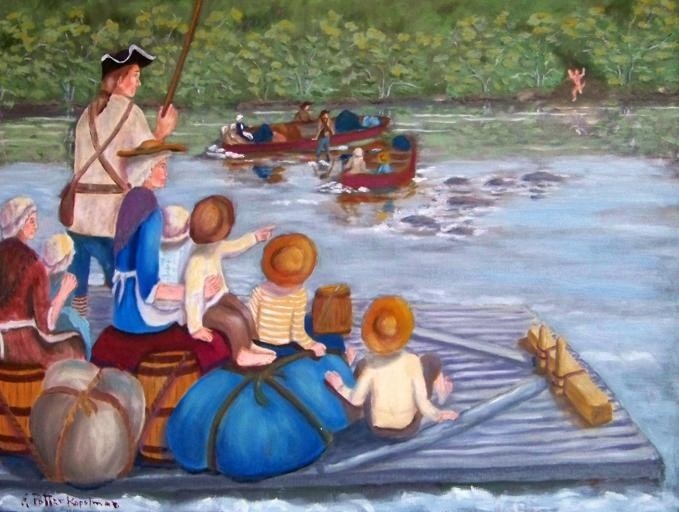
[
  {"x1": 234, "y1": 111, "x2": 275, "y2": 143},
  {"x1": 343, "y1": 144, "x2": 368, "y2": 174},
  {"x1": 182, "y1": 191, "x2": 280, "y2": 369},
  {"x1": 110, "y1": 137, "x2": 226, "y2": 336},
  {"x1": 157, "y1": 203, "x2": 195, "y2": 310},
  {"x1": 236, "y1": 155, "x2": 285, "y2": 185},
  {"x1": 303, "y1": 279, "x2": 357, "y2": 366},
  {"x1": 310, "y1": 109, "x2": 336, "y2": 165},
  {"x1": 311, "y1": 161, "x2": 336, "y2": 183},
  {"x1": 42, "y1": 230, "x2": 91, "y2": 364},
  {"x1": 368, "y1": 141, "x2": 396, "y2": 175},
  {"x1": 61, "y1": 41, "x2": 180, "y2": 317},
  {"x1": 1, "y1": 194, "x2": 91, "y2": 380},
  {"x1": 337, "y1": 201, "x2": 367, "y2": 225},
  {"x1": 295, "y1": 101, "x2": 316, "y2": 121},
  {"x1": 566, "y1": 65, "x2": 589, "y2": 104},
  {"x1": 242, "y1": 233, "x2": 329, "y2": 359},
  {"x1": 322, "y1": 292, "x2": 461, "y2": 443},
  {"x1": 376, "y1": 200, "x2": 395, "y2": 222}
]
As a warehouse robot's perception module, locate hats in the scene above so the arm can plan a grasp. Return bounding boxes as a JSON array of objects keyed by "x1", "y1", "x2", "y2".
[
  {"x1": 352, "y1": 146, "x2": 363, "y2": 157},
  {"x1": 261, "y1": 234, "x2": 316, "y2": 286},
  {"x1": 117, "y1": 140, "x2": 187, "y2": 157},
  {"x1": 319, "y1": 110, "x2": 330, "y2": 118},
  {"x1": 1, "y1": 196, "x2": 36, "y2": 239},
  {"x1": 361, "y1": 295, "x2": 414, "y2": 354},
  {"x1": 100, "y1": 43, "x2": 156, "y2": 80},
  {"x1": 161, "y1": 205, "x2": 191, "y2": 243},
  {"x1": 38, "y1": 233, "x2": 76, "y2": 274},
  {"x1": 300, "y1": 101, "x2": 313, "y2": 108},
  {"x1": 377, "y1": 152, "x2": 390, "y2": 164},
  {"x1": 188, "y1": 195, "x2": 235, "y2": 245},
  {"x1": 236, "y1": 114, "x2": 244, "y2": 121}
]
